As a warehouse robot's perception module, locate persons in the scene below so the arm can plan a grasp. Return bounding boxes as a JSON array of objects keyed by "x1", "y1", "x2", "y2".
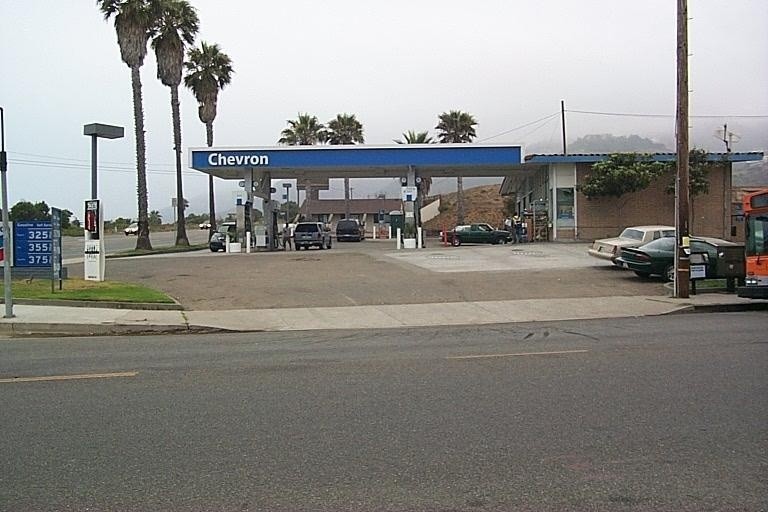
[
  {"x1": 282, "y1": 222, "x2": 292, "y2": 250},
  {"x1": 505, "y1": 215, "x2": 513, "y2": 232},
  {"x1": 511, "y1": 212, "x2": 523, "y2": 244}
]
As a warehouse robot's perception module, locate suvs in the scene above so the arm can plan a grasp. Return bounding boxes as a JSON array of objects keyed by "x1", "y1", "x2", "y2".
[
  {"x1": 294, "y1": 223, "x2": 332, "y2": 250},
  {"x1": 335, "y1": 219, "x2": 366, "y2": 242},
  {"x1": 124, "y1": 223, "x2": 139, "y2": 236}
]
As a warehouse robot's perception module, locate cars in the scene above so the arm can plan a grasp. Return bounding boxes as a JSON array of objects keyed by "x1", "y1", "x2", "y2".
[
  {"x1": 199, "y1": 221, "x2": 210, "y2": 230},
  {"x1": 587, "y1": 225, "x2": 736, "y2": 282},
  {"x1": 209, "y1": 221, "x2": 256, "y2": 253},
  {"x1": 439, "y1": 223, "x2": 512, "y2": 246}
]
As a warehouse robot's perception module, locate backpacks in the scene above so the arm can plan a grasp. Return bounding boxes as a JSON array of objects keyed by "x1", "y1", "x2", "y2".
[{"x1": 513, "y1": 217, "x2": 522, "y2": 229}]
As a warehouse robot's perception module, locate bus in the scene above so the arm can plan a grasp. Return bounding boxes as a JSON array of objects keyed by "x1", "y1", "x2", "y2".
[{"x1": 736, "y1": 185, "x2": 767, "y2": 300}]
